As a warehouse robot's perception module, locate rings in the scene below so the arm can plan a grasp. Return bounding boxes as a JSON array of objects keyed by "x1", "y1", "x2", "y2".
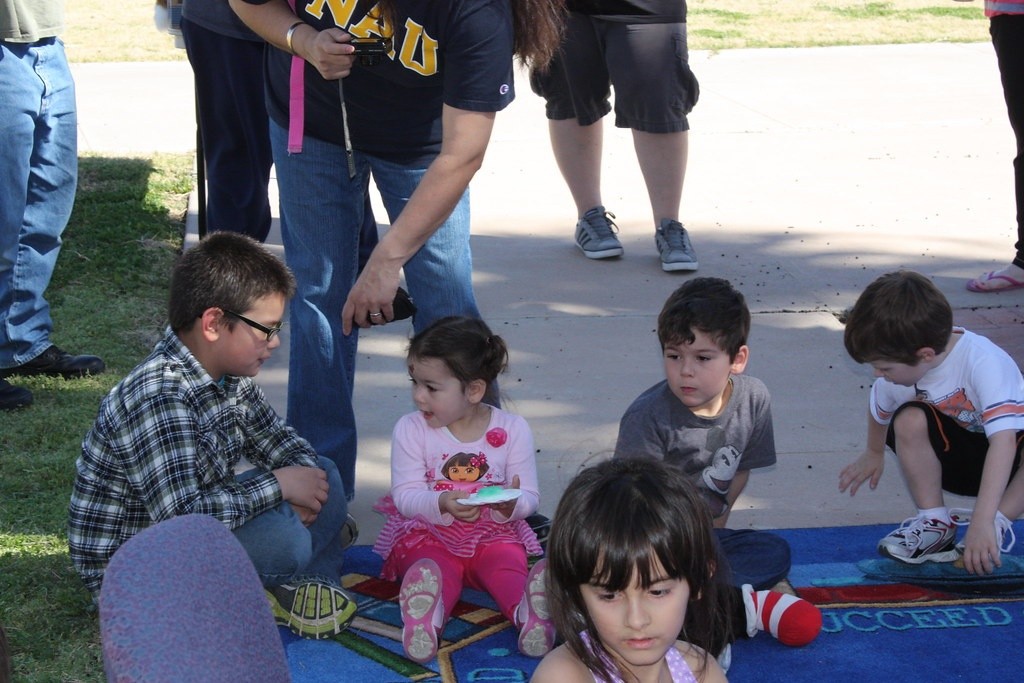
[{"x1": 370, "y1": 312, "x2": 380, "y2": 317}]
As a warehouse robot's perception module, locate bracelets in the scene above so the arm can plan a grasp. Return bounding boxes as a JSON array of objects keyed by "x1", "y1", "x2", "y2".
[{"x1": 287, "y1": 21, "x2": 306, "y2": 55}]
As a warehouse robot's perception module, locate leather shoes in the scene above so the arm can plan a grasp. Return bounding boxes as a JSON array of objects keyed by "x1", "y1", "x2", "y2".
[
  {"x1": 0, "y1": 379, "x2": 32, "y2": 407},
  {"x1": 0, "y1": 346, "x2": 105, "y2": 377}
]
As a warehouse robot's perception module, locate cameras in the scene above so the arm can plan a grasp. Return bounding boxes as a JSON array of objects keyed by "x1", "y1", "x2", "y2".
[{"x1": 342, "y1": 38, "x2": 393, "y2": 55}]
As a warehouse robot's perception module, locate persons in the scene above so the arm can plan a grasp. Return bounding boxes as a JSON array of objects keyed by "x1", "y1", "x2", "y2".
[
  {"x1": 966, "y1": 0, "x2": 1024, "y2": 293},
  {"x1": 228, "y1": 0, "x2": 572, "y2": 502},
  {"x1": 529, "y1": 0, "x2": 700, "y2": 272},
  {"x1": 67, "y1": 230, "x2": 360, "y2": 641},
  {"x1": 153, "y1": 1, "x2": 274, "y2": 244},
  {"x1": 0, "y1": 0, "x2": 106, "y2": 412},
  {"x1": 529, "y1": 457, "x2": 730, "y2": 683},
  {"x1": 613, "y1": 278, "x2": 821, "y2": 654},
  {"x1": 838, "y1": 270, "x2": 1024, "y2": 576},
  {"x1": 372, "y1": 317, "x2": 559, "y2": 663}
]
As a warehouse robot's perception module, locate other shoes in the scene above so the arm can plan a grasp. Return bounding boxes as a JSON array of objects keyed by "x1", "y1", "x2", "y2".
[
  {"x1": 338, "y1": 514, "x2": 359, "y2": 551},
  {"x1": 263, "y1": 576, "x2": 358, "y2": 641}
]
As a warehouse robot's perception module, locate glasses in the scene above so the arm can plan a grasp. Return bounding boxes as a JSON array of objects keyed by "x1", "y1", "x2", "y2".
[{"x1": 198, "y1": 309, "x2": 284, "y2": 343}]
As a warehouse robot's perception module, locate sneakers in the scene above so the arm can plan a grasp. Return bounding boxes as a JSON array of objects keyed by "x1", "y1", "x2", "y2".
[
  {"x1": 656, "y1": 216, "x2": 698, "y2": 272},
  {"x1": 513, "y1": 556, "x2": 561, "y2": 659},
  {"x1": 952, "y1": 513, "x2": 1015, "y2": 562},
  {"x1": 574, "y1": 206, "x2": 623, "y2": 260},
  {"x1": 398, "y1": 555, "x2": 446, "y2": 664},
  {"x1": 876, "y1": 513, "x2": 961, "y2": 565}
]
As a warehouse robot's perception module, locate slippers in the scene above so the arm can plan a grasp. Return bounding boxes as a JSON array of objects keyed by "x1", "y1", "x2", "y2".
[{"x1": 965, "y1": 267, "x2": 1024, "y2": 293}]
